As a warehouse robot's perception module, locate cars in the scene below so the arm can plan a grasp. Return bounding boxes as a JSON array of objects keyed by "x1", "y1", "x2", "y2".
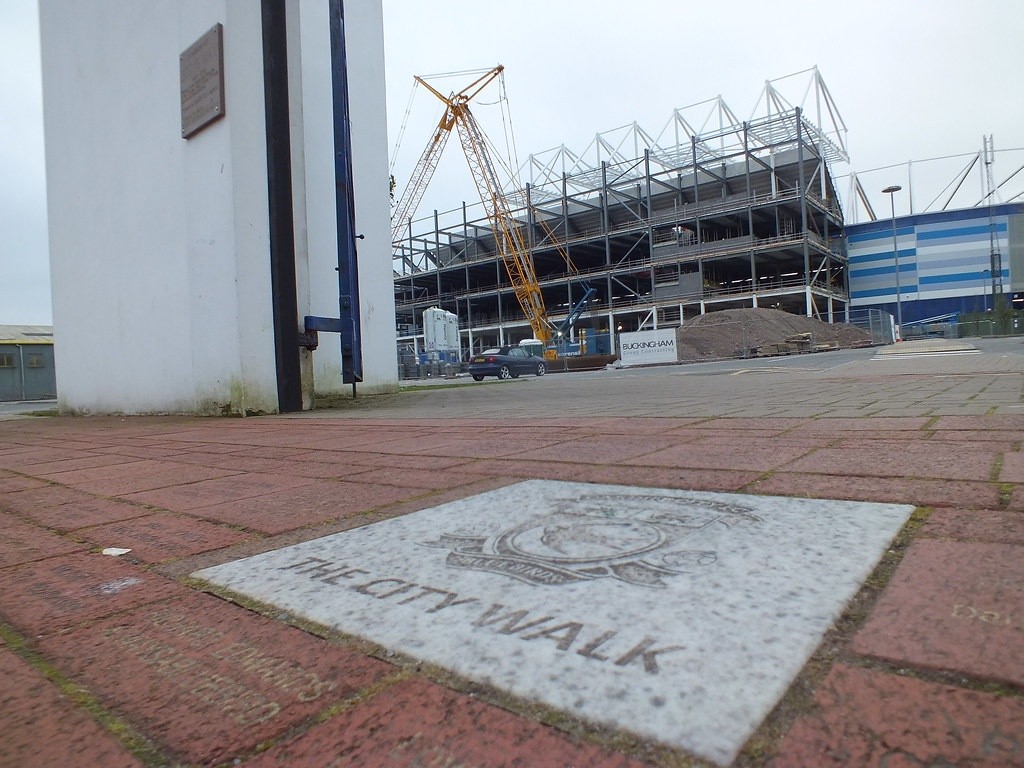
[{"x1": 467, "y1": 346, "x2": 549, "y2": 381}]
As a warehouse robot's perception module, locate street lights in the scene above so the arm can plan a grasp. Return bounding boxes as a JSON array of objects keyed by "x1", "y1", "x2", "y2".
[
  {"x1": 982, "y1": 269, "x2": 990, "y2": 314},
  {"x1": 882, "y1": 186, "x2": 902, "y2": 341}
]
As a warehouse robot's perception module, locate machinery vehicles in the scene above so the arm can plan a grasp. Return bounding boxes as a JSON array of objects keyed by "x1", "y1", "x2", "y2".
[{"x1": 387, "y1": 65, "x2": 597, "y2": 361}]
